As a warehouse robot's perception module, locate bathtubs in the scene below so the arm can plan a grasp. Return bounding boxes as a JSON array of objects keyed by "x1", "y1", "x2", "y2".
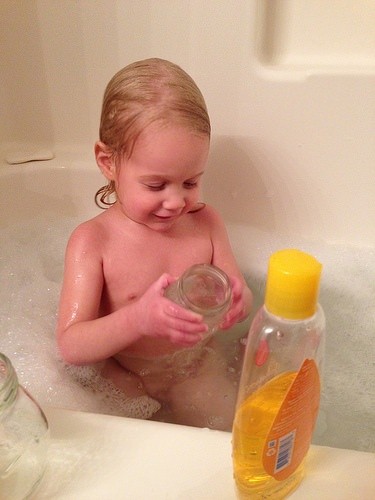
[{"x1": 1, "y1": 154, "x2": 375, "y2": 500}]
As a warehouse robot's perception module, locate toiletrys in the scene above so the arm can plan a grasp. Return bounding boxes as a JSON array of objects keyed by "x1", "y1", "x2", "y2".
[{"x1": 230, "y1": 248, "x2": 328, "y2": 500}]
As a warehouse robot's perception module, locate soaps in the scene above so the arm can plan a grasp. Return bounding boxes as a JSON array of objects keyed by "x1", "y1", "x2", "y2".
[{"x1": 5, "y1": 150, "x2": 54, "y2": 165}]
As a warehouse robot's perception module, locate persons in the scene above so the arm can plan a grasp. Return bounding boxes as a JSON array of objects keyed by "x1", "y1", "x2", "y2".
[{"x1": 53, "y1": 58, "x2": 255, "y2": 434}]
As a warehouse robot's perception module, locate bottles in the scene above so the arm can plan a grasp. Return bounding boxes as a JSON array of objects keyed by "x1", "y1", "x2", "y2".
[
  {"x1": 231, "y1": 248, "x2": 327, "y2": 500},
  {"x1": 146, "y1": 262, "x2": 235, "y2": 368},
  {"x1": 0, "y1": 355, "x2": 50, "y2": 500}
]
